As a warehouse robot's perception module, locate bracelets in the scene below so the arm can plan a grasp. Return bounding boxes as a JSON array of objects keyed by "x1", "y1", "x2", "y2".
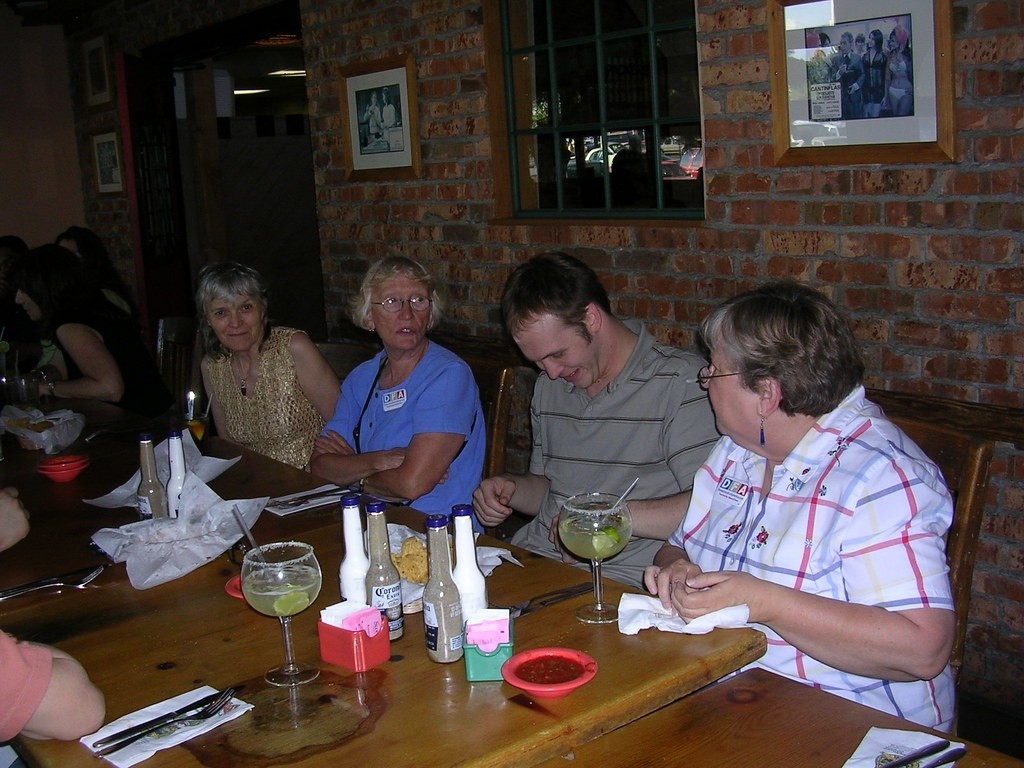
[
  {"x1": 358, "y1": 475, "x2": 366, "y2": 493},
  {"x1": 47, "y1": 379, "x2": 56, "y2": 398}
]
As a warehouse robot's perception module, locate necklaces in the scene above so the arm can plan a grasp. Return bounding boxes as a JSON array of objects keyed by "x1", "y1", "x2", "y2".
[{"x1": 232, "y1": 354, "x2": 253, "y2": 396}]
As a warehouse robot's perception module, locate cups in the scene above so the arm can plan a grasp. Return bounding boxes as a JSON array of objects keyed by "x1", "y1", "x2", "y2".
[
  {"x1": 1, "y1": 351, "x2": 6, "y2": 377},
  {"x1": 170, "y1": 413, "x2": 210, "y2": 458},
  {"x1": 9, "y1": 375, "x2": 40, "y2": 411}
]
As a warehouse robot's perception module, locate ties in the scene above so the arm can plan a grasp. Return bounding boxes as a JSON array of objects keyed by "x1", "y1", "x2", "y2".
[{"x1": 844, "y1": 55, "x2": 850, "y2": 69}]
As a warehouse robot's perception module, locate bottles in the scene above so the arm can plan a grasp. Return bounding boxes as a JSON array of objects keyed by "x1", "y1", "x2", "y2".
[
  {"x1": 452, "y1": 504, "x2": 487, "y2": 653},
  {"x1": 363, "y1": 502, "x2": 405, "y2": 641},
  {"x1": 338, "y1": 494, "x2": 369, "y2": 605},
  {"x1": 422, "y1": 513, "x2": 463, "y2": 663},
  {"x1": 166, "y1": 431, "x2": 194, "y2": 520},
  {"x1": 137, "y1": 433, "x2": 166, "y2": 521}
]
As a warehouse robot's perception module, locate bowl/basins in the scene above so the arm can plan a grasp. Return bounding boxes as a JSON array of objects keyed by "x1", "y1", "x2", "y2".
[{"x1": 36, "y1": 455, "x2": 89, "y2": 482}]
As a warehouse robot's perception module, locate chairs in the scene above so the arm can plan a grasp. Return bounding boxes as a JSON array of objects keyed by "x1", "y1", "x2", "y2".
[
  {"x1": 885, "y1": 413, "x2": 993, "y2": 736},
  {"x1": 449, "y1": 347, "x2": 516, "y2": 538},
  {"x1": 157, "y1": 318, "x2": 206, "y2": 416},
  {"x1": 314, "y1": 341, "x2": 379, "y2": 380}
]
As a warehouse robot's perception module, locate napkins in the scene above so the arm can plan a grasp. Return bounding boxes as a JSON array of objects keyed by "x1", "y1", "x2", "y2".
[
  {"x1": 78, "y1": 685, "x2": 255, "y2": 768},
  {"x1": 618, "y1": 590, "x2": 752, "y2": 637},
  {"x1": 840, "y1": 726, "x2": 966, "y2": 768},
  {"x1": 263, "y1": 483, "x2": 362, "y2": 517}
]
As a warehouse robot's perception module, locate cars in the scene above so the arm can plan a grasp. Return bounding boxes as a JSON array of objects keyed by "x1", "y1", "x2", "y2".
[{"x1": 528, "y1": 130, "x2": 704, "y2": 180}]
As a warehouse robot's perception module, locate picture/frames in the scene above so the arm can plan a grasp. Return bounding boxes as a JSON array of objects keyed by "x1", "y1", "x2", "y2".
[
  {"x1": 87, "y1": 125, "x2": 127, "y2": 199},
  {"x1": 335, "y1": 53, "x2": 423, "y2": 184},
  {"x1": 765, "y1": 0, "x2": 956, "y2": 166},
  {"x1": 77, "y1": 32, "x2": 116, "y2": 116}
]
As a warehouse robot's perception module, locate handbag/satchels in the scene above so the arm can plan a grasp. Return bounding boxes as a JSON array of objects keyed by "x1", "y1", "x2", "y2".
[{"x1": 845, "y1": 71, "x2": 861, "y2": 104}]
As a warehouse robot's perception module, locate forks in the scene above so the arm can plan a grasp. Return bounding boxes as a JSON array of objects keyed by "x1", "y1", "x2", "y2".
[
  {"x1": 0, "y1": 566, "x2": 103, "y2": 601},
  {"x1": 274, "y1": 491, "x2": 358, "y2": 506},
  {"x1": 489, "y1": 582, "x2": 594, "y2": 610},
  {"x1": 93, "y1": 687, "x2": 239, "y2": 758}
]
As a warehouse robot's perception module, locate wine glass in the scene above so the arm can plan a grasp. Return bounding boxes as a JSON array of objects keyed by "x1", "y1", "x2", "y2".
[
  {"x1": 557, "y1": 493, "x2": 633, "y2": 624},
  {"x1": 240, "y1": 541, "x2": 322, "y2": 686}
]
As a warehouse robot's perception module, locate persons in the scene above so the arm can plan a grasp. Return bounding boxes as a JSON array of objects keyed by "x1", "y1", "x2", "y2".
[
  {"x1": 470, "y1": 248, "x2": 722, "y2": 594},
  {"x1": 0, "y1": 480, "x2": 106, "y2": 768},
  {"x1": 647, "y1": 276, "x2": 960, "y2": 733},
  {"x1": 816, "y1": 25, "x2": 912, "y2": 122},
  {"x1": 305, "y1": 252, "x2": 489, "y2": 538},
  {"x1": 363, "y1": 86, "x2": 396, "y2": 143},
  {"x1": 192, "y1": 254, "x2": 345, "y2": 474},
  {"x1": 0, "y1": 225, "x2": 155, "y2": 412}
]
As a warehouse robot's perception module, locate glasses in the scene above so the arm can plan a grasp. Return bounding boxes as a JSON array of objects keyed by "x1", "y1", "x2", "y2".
[
  {"x1": 697, "y1": 361, "x2": 741, "y2": 383},
  {"x1": 370, "y1": 295, "x2": 432, "y2": 312}
]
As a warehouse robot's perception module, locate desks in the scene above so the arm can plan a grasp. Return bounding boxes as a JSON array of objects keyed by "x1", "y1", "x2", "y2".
[
  {"x1": 528, "y1": 666, "x2": 1023, "y2": 768},
  {"x1": 0, "y1": 399, "x2": 769, "y2": 768}
]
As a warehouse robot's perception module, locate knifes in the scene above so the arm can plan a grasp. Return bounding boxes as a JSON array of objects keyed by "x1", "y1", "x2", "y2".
[
  {"x1": 509, "y1": 587, "x2": 594, "y2": 618},
  {"x1": 0, "y1": 563, "x2": 112, "y2": 595},
  {"x1": 93, "y1": 684, "x2": 246, "y2": 748},
  {"x1": 262, "y1": 486, "x2": 349, "y2": 508}
]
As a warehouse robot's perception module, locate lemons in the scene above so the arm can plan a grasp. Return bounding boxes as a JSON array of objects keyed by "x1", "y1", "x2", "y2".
[
  {"x1": 274, "y1": 589, "x2": 310, "y2": 615},
  {"x1": 591, "y1": 526, "x2": 620, "y2": 553},
  {"x1": 193, "y1": 420, "x2": 205, "y2": 440}
]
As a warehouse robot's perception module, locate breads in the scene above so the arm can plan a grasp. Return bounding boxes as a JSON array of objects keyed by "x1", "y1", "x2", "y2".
[
  {"x1": 7, "y1": 417, "x2": 54, "y2": 433},
  {"x1": 390, "y1": 537, "x2": 428, "y2": 584}
]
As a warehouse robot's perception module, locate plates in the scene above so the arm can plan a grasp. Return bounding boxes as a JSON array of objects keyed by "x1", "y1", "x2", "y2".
[
  {"x1": 500, "y1": 647, "x2": 598, "y2": 695},
  {"x1": 225, "y1": 574, "x2": 245, "y2": 600}
]
